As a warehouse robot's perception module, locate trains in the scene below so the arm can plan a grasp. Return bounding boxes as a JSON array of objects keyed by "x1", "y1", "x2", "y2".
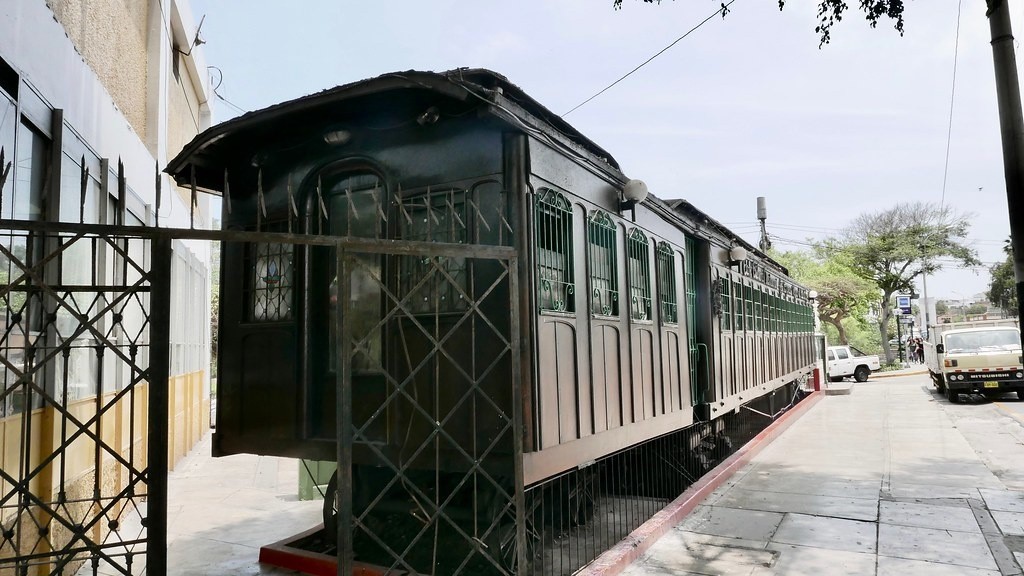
[{"x1": 160, "y1": 64, "x2": 823, "y2": 550}]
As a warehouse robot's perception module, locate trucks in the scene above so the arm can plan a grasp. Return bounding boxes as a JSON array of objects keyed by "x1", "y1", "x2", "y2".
[{"x1": 920, "y1": 319, "x2": 1024, "y2": 404}]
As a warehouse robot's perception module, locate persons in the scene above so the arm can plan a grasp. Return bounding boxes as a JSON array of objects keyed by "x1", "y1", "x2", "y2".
[{"x1": 908, "y1": 338, "x2": 924, "y2": 364}]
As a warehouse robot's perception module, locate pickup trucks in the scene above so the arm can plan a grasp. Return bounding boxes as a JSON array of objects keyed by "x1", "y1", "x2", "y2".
[{"x1": 826, "y1": 345, "x2": 882, "y2": 382}]
